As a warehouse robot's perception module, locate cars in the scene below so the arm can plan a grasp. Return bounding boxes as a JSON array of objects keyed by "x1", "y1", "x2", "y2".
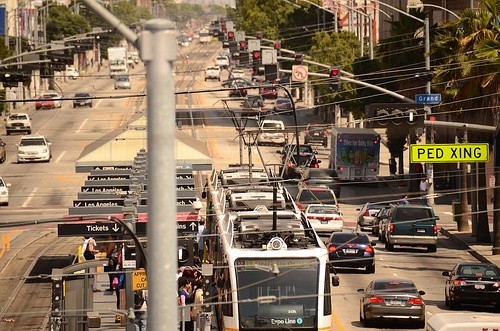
[
  {"x1": 441, "y1": 259, "x2": 500, "y2": 311},
  {"x1": 357, "y1": 202, "x2": 439, "y2": 253},
  {"x1": 114, "y1": 76, "x2": 131, "y2": 90},
  {"x1": 326, "y1": 231, "x2": 377, "y2": 274},
  {"x1": 355, "y1": 279, "x2": 426, "y2": 329},
  {"x1": 297, "y1": 168, "x2": 340, "y2": 198},
  {"x1": 15, "y1": 136, "x2": 52, "y2": 162},
  {"x1": 276, "y1": 144, "x2": 320, "y2": 181},
  {"x1": 35, "y1": 90, "x2": 62, "y2": 111},
  {"x1": 72, "y1": 93, "x2": 92, "y2": 106},
  {"x1": 0, "y1": 177, "x2": 11, "y2": 206},
  {"x1": 67, "y1": 68, "x2": 78, "y2": 80},
  {"x1": 295, "y1": 188, "x2": 339, "y2": 216},
  {"x1": 0, "y1": 138, "x2": 7, "y2": 163},
  {"x1": 303, "y1": 203, "x2": 344, "y2": 236},
  {"x1": 178, "y1": 20, "x2": 237, "y2": 68},
  {"x1": 3, "y1": 113, "x2": 32, "y2": 134},
  {"x1": 221, "y1": 69, "x2": 327, "y2": 147},
  {"x1": 204, "y1": 66, "x2": 221, "y2": 81}
]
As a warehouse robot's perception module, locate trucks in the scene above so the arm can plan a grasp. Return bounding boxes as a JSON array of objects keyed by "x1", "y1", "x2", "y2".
[
  {"x1": 107, "y1": 46, "x2": 129, "y2": 79},
  {"x1": 327, "y1": 127, "x2": 381, "y2": 184},
  {"x1": 122, "y1": 39, "x2": 139, "y2": 68}
]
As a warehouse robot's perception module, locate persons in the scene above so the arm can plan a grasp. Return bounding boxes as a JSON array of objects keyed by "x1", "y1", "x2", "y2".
[
  {"x1": 389, "y1": 157, "x2": 397, "y2": 175},
  {"x1": 399, "y1": 195, "x2": 409, "y2": 204},
  {"x1": 177, "y1": 258, "x2": 206, "y2": 331},
  {"x1": 420, "y1": 176, "x2": 428, "y2": 192},
  {"x1": 101, "y1": 236, "x2": 126, "y2": 323},
  {"x1": 82, "y1": 236, "x2": 99, "y2": 292}
]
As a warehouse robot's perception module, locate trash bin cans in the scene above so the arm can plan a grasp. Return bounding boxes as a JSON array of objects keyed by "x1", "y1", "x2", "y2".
[
  {"x1": 389, "y1": 159, "x2": 397, "y2": 173},
  {"x1": 452, "y1": 201, "x2": 461, "y2": 222}
]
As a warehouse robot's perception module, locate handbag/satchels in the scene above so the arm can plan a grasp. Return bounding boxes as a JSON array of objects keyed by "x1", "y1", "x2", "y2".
[{"x1": 84, "y1": 243, "x2": 95, "y2": 260}]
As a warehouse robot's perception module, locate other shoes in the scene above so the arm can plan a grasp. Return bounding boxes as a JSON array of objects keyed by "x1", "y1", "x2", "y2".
[
  {"x1": 106, "y1": 289, "x2": 114, "y2": 291},
  {"x1": 93, "y1": 289, "x2": 101, "y2": 292}
]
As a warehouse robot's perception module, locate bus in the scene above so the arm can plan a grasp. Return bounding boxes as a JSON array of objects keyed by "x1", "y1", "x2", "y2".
[{"x1": 208, "y1": 166, "x2": 340, "y2": 331}]
